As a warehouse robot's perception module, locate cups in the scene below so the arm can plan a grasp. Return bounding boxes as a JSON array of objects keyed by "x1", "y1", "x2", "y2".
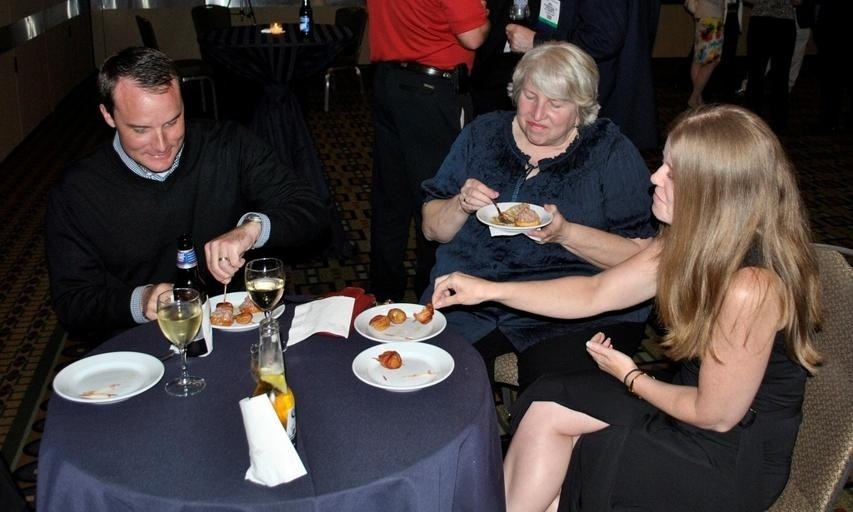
[{"x1": 509, "y1": 5, "x2": 530, "y2": 25}]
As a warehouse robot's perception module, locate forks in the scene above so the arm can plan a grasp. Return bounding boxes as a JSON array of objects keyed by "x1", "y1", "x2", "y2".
[{"x1": 491, "y1": 194, "x2": 517, "y2": 224}]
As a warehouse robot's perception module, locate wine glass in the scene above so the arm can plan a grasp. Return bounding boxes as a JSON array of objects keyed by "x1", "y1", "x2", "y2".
[
  {"x1": 244, "y1": 255, "x2": 288, "y2": 355},
  {"x1": 156, "y1": 287, "x2": 207, "y2": 398}
]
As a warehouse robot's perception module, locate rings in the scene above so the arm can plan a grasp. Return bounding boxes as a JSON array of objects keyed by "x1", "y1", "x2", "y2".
[
  {"x1": 217, "y1": 254, "x2": 230, "y2": 264},
  {"x1": 461, "y1": 194, "x2": 469, "y2": 204}
]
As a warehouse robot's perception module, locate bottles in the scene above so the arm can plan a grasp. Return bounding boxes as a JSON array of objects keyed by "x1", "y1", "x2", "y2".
[
  {"x1": 170, "y1": 233, "x2": 213, "y2": 355},
  {"x1": 249, "y1": 316, "x2": 298, "y2": 451},
  {"x1": 297, "y1": 0, "x2": 313, "y2": 40}
]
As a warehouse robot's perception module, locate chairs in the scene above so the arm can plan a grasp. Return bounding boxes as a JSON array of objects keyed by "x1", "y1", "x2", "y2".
[
  {"x1": 191, "y1": 5, "x2": 233, "y2": 58},
  {"x1": 324, "y1": 8, "x2": 371, "y2": 112},
  {"x1": 763, "y1": 245, "x2": 851, "y2": 512},
  {"x1": 135, "y1": 15, "x2": 222, "y2": 122}
]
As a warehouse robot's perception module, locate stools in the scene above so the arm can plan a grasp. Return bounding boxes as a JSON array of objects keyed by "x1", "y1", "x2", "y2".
[{"x1": 487, "y1": 349, "x2": 523, "y2": 411}]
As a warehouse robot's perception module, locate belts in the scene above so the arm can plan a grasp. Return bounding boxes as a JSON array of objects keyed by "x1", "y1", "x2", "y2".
[{"x1": 401, "y1": 59, "x2": 451, "y2": 79}]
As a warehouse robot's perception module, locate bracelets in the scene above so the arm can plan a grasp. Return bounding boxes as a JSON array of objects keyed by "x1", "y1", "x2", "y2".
[
  {"x1": 623, "y1": 369, "x2": 642, "y2": 386},
  {"x1": 629, "y1": 371, "x2": 647, "y2": 392}
]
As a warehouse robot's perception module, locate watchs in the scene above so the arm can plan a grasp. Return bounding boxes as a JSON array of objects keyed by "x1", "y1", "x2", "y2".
[{"x1": 243, "y1": 214, "x2": 261, "y2": 226}]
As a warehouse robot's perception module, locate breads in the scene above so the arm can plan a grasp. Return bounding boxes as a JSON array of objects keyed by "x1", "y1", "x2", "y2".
[
  {"x1": 498, "y1": 202, "x2": 540, "y2": 226},
  {"x1": 414, "y1": 304, "x2": 434, "y2": 324},
  {"x1": 369, "y1": 315, "x2": 390, "y2": 332},
  {"x1": 211, "y1": 296, "x2": 261, "y2": 325},
  {"x1": 388, "y1": 308, "x2": 406, "y2": 324},
  {"x1": 379, "y1": 350, "x2": 402, "y2": 369}
]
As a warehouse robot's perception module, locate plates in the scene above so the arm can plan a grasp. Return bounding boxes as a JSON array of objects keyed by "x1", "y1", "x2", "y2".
[
  {"x1": 352, "y1": 302, "x2": 448, "y2": 344},
  {"x1": 351, "y1": 342, "x2": 457, "y2": 394},
  {"x1": 475, "y1": 201, "x2": 555, "y2": 231},
  {"x1": 50, "y1": 351, "x2": 165, "y2": 406},
  {"x1": 202, "y1": 291, "x2": 285, "y2": 332}
]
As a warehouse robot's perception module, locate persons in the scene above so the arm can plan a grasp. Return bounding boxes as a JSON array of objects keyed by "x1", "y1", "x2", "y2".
[
  {"x1": 685, "y1": 0, "x2": 852, "y2": 146},
  {"x1": 471, "y1": 0, "x2": 541, "y2": 117},
  {"x1": 433, "y1": 101, "x2": 823, "y2": 512},
  {"x1": 361, "y1": 0, "x2": 492, "y2": 306},
  {"x1": 42, "y1": 45, "x2": 329, "y2": 354},
  {"x1": 496, "y1": 0, "x2": 653, "y2": 169},
  {"x1": 415, "y1": 39, "x2": 656, "y2": 399}
]
